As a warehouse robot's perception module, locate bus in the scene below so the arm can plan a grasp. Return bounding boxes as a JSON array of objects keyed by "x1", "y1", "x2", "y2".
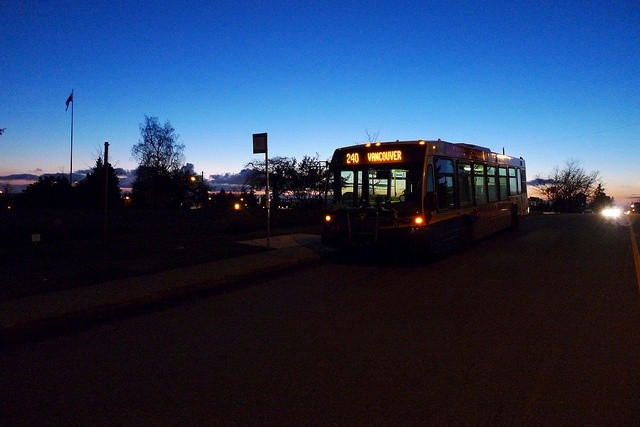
[{"x1": 318, "y1": 139, "x2": 531, "y2": 267}]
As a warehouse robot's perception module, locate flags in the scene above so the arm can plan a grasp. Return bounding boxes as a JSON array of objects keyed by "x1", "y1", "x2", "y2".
[{"x1": 65, "y1": 88, "x2": 74, "y2": 111}]
